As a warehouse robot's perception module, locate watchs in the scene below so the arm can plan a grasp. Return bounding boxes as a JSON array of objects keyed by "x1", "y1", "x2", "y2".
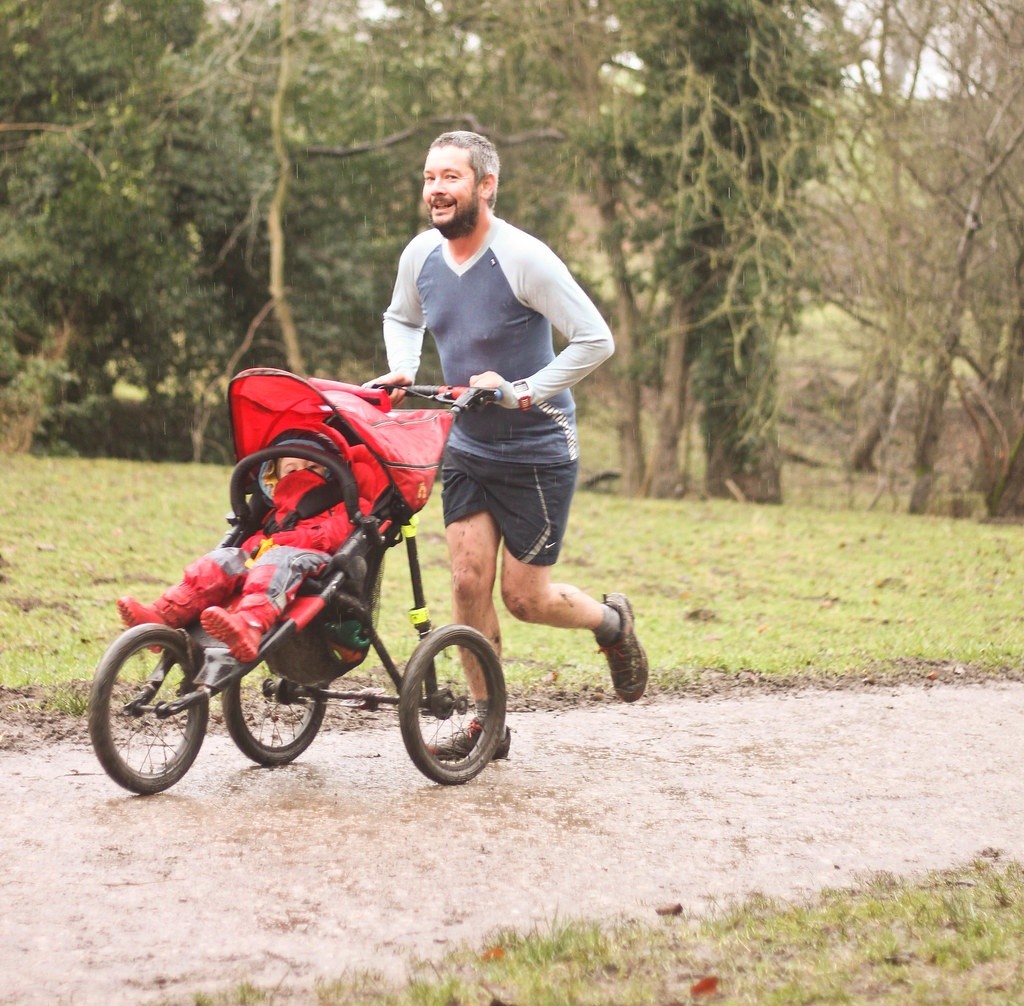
[{"x1": 513, "y1": 379, "x2": 534, "y2": 410}]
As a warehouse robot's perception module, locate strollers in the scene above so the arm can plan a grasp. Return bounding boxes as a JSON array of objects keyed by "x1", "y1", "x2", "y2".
[{"x1": 86, "y1": 364, "x2": 504, "y2": 793}]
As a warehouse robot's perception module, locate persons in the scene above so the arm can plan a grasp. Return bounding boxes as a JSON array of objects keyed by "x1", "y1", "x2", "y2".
[
  {"x1": 117, "y1": 458, "x2": 373, "y2": 662},
  {"x1": 363, "y1": 131, "x2": 649, "y2": 759}
]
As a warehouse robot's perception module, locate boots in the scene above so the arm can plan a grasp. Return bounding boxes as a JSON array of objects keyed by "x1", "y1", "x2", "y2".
[
  {"x1": 116, "y1": 582, "x2": 206, "y2": 655},
  {"x1": 200, "y1": 594, "x2": 277, "y2": 664}
]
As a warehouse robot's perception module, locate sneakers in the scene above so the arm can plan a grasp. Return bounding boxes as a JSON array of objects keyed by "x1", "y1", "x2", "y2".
[
  {"x1": 426, "y1": 720, "x2": 514, "y2": 761},
  {"x1": 592, "y1": 593, "x2": 647, "y2": 702}
]
